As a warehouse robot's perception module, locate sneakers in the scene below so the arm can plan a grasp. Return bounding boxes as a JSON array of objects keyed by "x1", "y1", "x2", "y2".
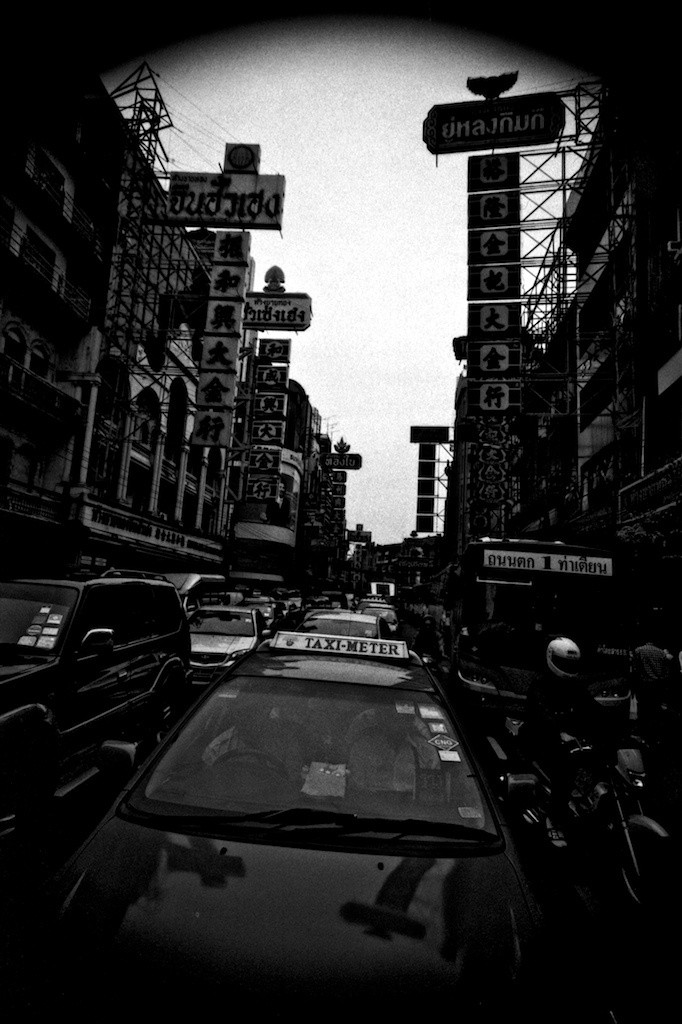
[{"x1": 546, "y1": 813, "x2": 567, "y2": 849}]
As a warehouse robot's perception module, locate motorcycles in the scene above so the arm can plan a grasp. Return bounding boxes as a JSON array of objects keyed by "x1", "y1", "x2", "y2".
[{"x1": 494, "y1": 717, "x2": 674, "y2": 909}]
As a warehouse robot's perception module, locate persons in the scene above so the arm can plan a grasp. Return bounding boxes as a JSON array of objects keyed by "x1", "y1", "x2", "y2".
[
  {"x1": 518, "y1": 636, "x2": 650, "y2": 853},
  {"x1": 662, "y1": 646, "x2": 682, "y2": 717},
  {"x1": 271, "y1": 601, "x2": 311, "y2": 634},
  {"x1": 628, "y1": 636, "x2": 669, "y2": 747},
  {"x1": 412, "y1": 614, "x2": 450, "y2": 663}
]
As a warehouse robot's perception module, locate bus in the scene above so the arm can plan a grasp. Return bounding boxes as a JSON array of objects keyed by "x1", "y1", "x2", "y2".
[{"x1": 402, "y1": 535, "x2": 616, "y2": 712}]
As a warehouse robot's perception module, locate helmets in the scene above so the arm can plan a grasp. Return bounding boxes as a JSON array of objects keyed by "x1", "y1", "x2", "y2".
[{"x1": 546, "y1": 636, "x2": 581, "y2": 679}]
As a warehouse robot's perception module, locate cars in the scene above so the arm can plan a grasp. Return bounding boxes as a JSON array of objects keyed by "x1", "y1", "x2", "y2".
[{"x1": 0, "y1": 569, "x2": 528, "y2": 1024}]
